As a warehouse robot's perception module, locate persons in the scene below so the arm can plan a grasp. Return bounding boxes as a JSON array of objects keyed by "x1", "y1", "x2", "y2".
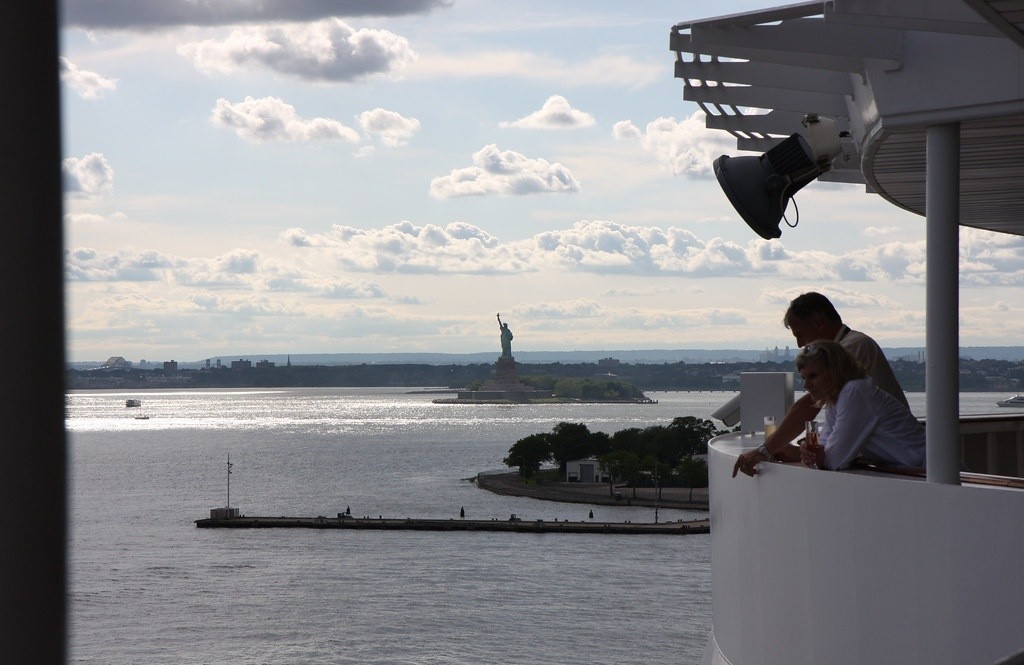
[
  {"x1": 796, "y1": 339, "x2": 969, "y2": 472},
  {"x1": 733, "y1": 293, "x2": 911, "y2": 478},
  {"x1": 497, "y1": 316, "x2": 513, "y2": 357}
]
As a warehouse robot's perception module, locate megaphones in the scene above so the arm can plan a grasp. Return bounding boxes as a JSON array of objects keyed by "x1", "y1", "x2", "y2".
[{"x1": 712, "y1": 114, "x2": 844, "y2": 239}]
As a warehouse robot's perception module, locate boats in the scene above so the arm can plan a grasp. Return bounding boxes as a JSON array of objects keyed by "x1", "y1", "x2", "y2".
[
  {"x1": 134, "y1": 412, "x2": 149, "y2": 419},
  {"x1": 125, "y1": 399, "x2": 142, "y2": 408},
  {"x1": 995, "y1": 394, "x2": 1024, "y2": 408}
]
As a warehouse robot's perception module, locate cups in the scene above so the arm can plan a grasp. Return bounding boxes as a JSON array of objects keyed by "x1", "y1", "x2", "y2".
[{"x1": 764, "y1": 416, "x2": 777, "y2": 442}]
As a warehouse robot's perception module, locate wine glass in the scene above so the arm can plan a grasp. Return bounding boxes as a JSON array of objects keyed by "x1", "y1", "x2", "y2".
[{"x1": 805, "y1": 421, "x2": 818, "y2": 469}]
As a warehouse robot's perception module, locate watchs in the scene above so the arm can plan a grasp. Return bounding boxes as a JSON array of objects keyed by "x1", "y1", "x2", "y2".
[{"x1": 759, "y1": 445, "x2": 771, "y2": 458}]
